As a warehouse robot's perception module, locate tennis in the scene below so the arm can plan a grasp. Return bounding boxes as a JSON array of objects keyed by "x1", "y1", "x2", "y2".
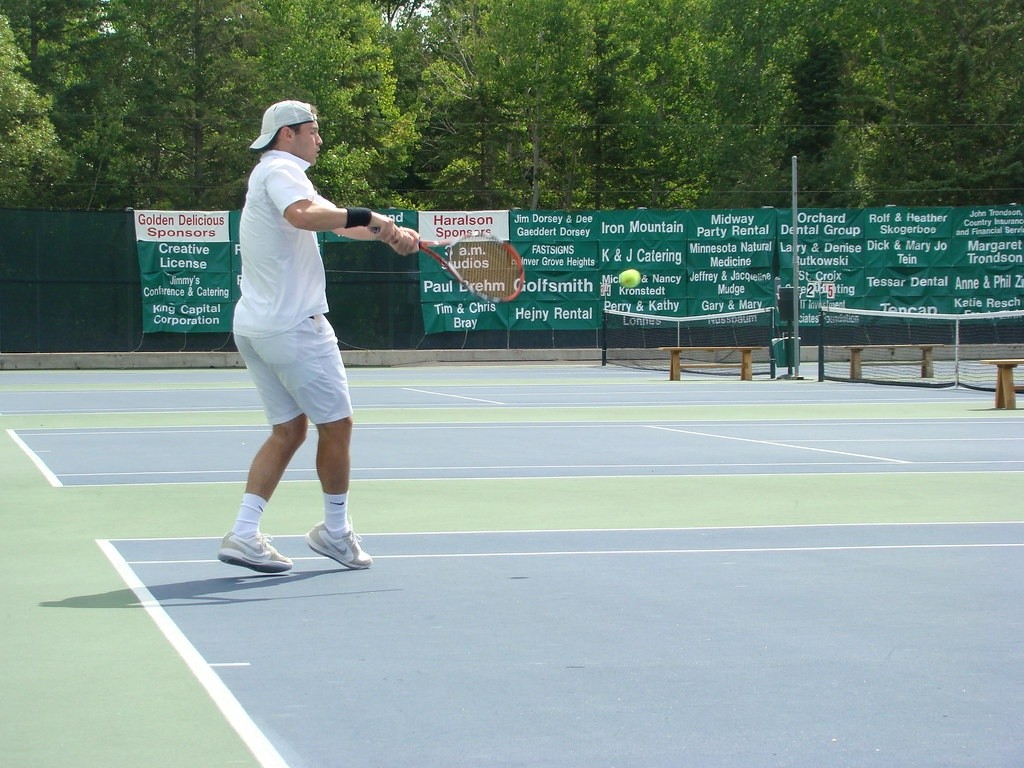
[{"x1": 618, "y1": 268, "x2": 641, "y2": 288}]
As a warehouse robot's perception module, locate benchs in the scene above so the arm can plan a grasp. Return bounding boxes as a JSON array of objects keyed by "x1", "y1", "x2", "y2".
[
  {"x1": 844, "y1": 344, "x2": 945, "y2": 379},
  {"x1": 979, "y1": 359, "x2": 1024, "y2": 410},
  {"x1": 658, "y1": 346, "x2": 763, "y2": 380}
]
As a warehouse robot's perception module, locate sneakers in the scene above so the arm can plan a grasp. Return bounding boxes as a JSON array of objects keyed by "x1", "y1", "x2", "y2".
[
  {"x1": 306, "y1": 516, "x2": 373, "y2": 570},
  {"x1": 218, "y1": 531, "x2": 293, "y2": 573}
]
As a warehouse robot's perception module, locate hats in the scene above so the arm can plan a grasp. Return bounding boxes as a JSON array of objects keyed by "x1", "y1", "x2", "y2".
[{"x1": 249, "y1": 100, "x2": 317, "y2": 149}]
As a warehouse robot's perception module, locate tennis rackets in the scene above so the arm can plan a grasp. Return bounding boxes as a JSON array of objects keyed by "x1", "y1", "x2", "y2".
[{"x1": 372, "y1": 226, "x2": 525, "y2": 302}]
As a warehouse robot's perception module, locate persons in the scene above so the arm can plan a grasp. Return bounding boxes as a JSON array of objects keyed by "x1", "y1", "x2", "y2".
[{"x1": 217, "y1": 99, "x2": 423, "y2": 573}]
